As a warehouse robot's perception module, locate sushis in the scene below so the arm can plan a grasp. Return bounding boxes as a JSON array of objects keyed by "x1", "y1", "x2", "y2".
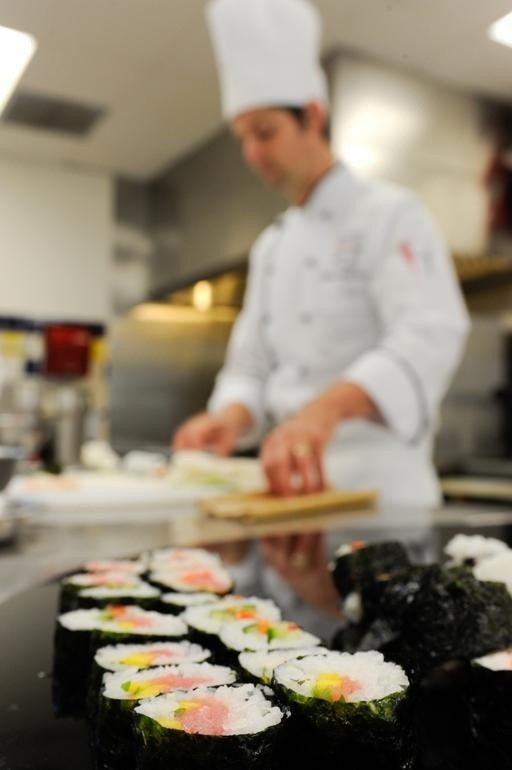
[
  {"x1": 326, "y1": 534, "x2": 512, "y2": 767},
  {"x1": 51, "y1": 534, "x2": 411, "y2": 770}
]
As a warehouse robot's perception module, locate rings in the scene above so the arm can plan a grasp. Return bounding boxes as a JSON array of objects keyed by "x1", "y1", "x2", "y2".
[
  {"x1": 290, "y1": 441, "x2": 315, "y2": 457},
  {"x1": 286, "y1": 550, "x2": 309, "y2": 574}
]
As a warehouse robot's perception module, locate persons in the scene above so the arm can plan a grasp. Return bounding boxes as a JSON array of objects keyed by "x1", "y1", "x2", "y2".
[
  {"x1": 170, "y1": 2, "x2": 475, "y2": 505},
  {"x1": 211, "y1": 532, "x2": 347, "y2": 642}
]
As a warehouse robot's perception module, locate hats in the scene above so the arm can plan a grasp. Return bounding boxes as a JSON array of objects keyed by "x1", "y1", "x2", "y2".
[{"x1": 206, "y1": 0, "x2": 331, "y2": 124}]
